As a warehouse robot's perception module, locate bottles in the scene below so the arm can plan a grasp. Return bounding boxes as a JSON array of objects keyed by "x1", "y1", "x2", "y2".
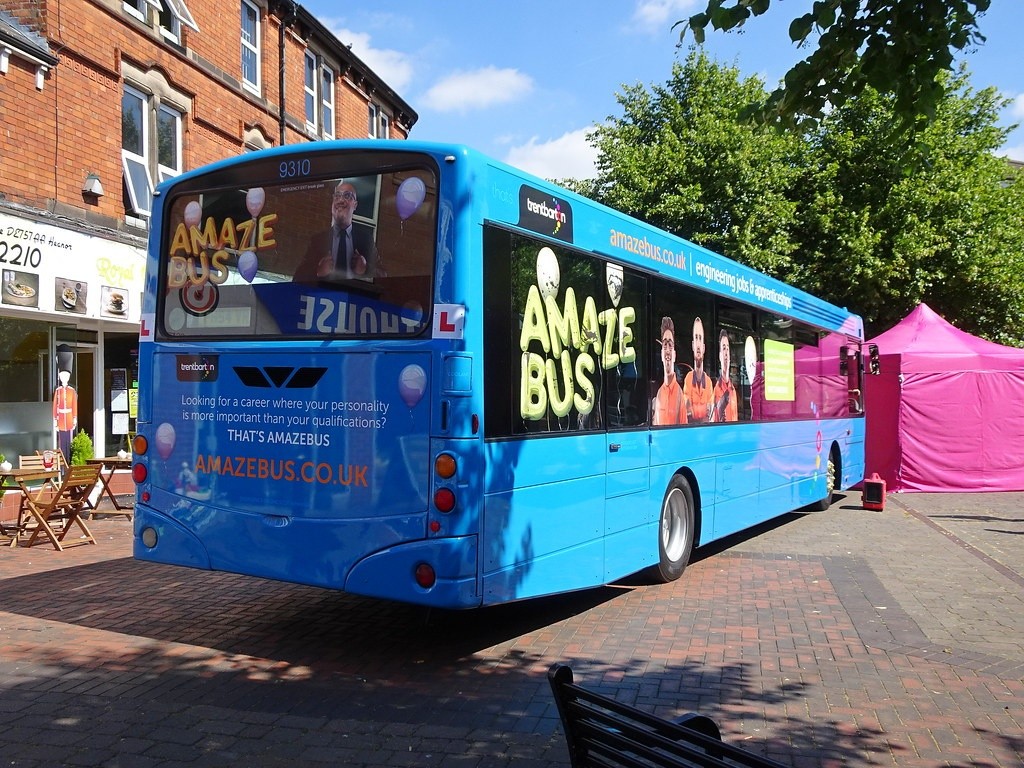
[{"x1": 42, "y1": 450, "x2": 54, "y2": 472}]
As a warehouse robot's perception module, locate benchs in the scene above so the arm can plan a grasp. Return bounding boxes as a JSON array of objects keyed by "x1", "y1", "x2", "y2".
[{"x1": 548, "y1": 663, "x2": 793, "y2": 768}]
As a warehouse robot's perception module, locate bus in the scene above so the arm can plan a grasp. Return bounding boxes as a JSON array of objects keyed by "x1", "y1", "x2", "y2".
[{"x1": 132, "y1": 136, "x2": 881, "y2": 615}]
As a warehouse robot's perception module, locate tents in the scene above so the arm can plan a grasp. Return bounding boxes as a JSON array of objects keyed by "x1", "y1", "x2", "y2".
[
  {"x1": 749, "y1": 317, "x2": 857, "y2": 423},
  {"x1": 862, "y1": 304, "x2": 1024, "y2": 492}
]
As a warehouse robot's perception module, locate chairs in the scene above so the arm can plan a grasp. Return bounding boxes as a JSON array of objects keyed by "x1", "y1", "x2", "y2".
[
  {"x1": 26, "y1": 462, "x2": 103, "y2": 551},
  {"x1": 36, "y1": 447, "x2": 98, "y2": 520},
  {"x1": 606, "y1": 355, "x2": 717, "y2": 427},
  {"x1": 18, "y1": 454, "x2": 66, "y2": 531}
]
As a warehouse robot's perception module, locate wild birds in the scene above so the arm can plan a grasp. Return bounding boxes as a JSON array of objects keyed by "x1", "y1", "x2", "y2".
[{"x1": 345, "y1": 42, "x2": 353, "y2": 50}]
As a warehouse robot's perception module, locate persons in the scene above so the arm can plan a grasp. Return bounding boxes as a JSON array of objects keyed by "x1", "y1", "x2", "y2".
[
  {"x1": 298, "y1": 183, "x2": 388, "y2": 299},
  {"x1": 653, "y1": 314, "x2": 741, "y2": 426}
]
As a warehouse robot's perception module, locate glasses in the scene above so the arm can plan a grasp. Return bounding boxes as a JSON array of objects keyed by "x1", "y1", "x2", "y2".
[{"x1": 333, "y1": 190, "x2": 354, "y2": 201}]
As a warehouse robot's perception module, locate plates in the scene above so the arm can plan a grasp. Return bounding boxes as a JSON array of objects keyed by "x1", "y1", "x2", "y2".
[
  {"x1": 7, "y1": 285, "x2": 36, "y2": 298},
  {"x1": 107, "y1": 300, "x2": 128, "y2": 314},
  {"x1": 61, "y1": 288, "x2": 77, "y2": 306}
]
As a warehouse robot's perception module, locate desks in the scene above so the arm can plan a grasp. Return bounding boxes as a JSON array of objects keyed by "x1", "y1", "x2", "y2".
[
  {"x1": 0, "y1": 469, "x2": 63, "y2": 548},
  {"x1": 85, "y1": 456, "x2": 134, "y2": 520}
]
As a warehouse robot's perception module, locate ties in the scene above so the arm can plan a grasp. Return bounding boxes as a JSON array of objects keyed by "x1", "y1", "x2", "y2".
[{"x1": 334, "y1": 230, "x2": 347, "y2": 279}]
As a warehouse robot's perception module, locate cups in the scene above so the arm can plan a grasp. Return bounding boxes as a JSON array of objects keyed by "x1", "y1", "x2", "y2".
[
  {"x1": 1, "y1": 460, "x2": 12, "y2": 473},
  {"x1": 117, "y1": 450, "x2": 126, "y2": 459}
]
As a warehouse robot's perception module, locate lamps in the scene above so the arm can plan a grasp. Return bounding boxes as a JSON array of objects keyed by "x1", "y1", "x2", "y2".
[{"x1": 81, "y1": 173, "x2": 105, "y2": 197}]
